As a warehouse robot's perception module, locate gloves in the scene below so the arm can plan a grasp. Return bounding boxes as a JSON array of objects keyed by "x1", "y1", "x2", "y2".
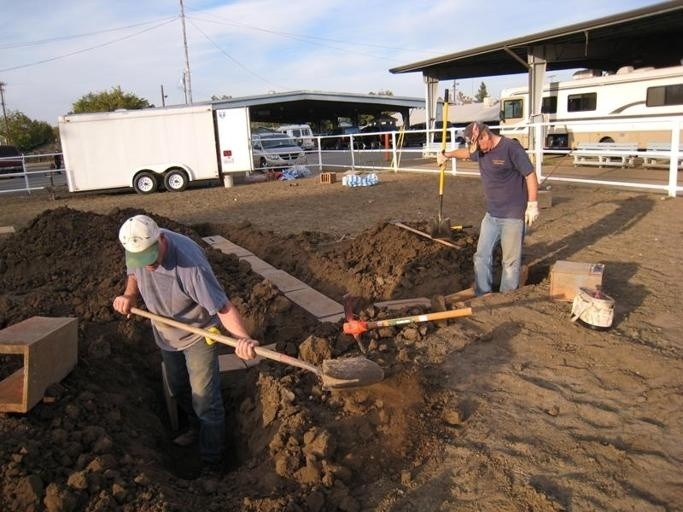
[
  {"x1": 524, "y1": 201, "x2": 540, "y2": 227},
  {"x1": 437, "y1": 148, "x2": 449, "y2": 172}
]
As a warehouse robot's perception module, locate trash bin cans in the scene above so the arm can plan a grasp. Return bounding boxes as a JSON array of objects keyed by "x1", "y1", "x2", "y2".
[{"x1": 223, "y1": 174, "x2": 234, "y2": 188}]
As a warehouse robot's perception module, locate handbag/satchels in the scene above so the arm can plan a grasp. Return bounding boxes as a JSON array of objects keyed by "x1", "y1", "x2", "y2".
[{"x1": 568, "y1": 286, "x2": 615, "y2": 330}]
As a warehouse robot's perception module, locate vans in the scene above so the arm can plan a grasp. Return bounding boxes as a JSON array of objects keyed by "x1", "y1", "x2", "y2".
[{"x1": 272, "y1": 124, "x2": 315, "y2": 150}]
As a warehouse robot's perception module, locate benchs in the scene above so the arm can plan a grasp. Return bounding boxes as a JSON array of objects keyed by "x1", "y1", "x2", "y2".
[
  {"x1": 641, "y1": 142, "x2": 683, "y2": 170},
  {"x1": 568, "y1": 142, "x2": 638, "y2": 168}
]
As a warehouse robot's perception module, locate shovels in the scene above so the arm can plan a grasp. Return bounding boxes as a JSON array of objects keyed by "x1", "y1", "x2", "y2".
[
  {"x1": 129, "y1": 306, "x2": 385, "y2": 392},
  {"x1": 431, "y1": 89, "x2": 453, "y2": 238}
]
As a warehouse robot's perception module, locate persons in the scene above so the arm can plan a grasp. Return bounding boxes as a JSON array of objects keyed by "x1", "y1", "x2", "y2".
[
  {"x1": 437, "y1": 121, "x2": 540, "y2": 296},
  {"x1": 53, "y1": 149, "x2": 61, "y2": 174},
  {"x1": 112, "y1": 214, "x2": 260, "y2": 482}
]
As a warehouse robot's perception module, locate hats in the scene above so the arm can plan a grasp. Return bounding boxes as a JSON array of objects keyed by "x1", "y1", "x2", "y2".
[
  {"x1": 119, "y1": 214, "x2": 162, "y2": 269},
  {"x1": 463, "y1": 121, "x2": 485, "y2": 153}
]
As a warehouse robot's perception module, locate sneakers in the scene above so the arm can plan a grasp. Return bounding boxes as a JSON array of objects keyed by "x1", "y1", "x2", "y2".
[
  {"x1": 171, "y1": 425, "x2": 202, "y2": 445},
  {"x1": 201, "y1": 468, "x2": 219, "y2": 494}
]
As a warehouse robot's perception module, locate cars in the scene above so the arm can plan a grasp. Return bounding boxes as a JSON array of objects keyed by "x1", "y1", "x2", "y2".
[
  {"x1": 324, "y1": 126, "x2": 363, "y2": 148},
  {"x1": 252, "y1": 132, "x2": 305, "y2": 173},
  {"x1": 0, "y1": 144, "x2": 24, "y2": 178}
]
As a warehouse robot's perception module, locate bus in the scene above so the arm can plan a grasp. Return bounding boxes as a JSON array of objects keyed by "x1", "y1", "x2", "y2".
[{"x1": 498, "y1": 66, "x2": 682, "y2": 154}]
